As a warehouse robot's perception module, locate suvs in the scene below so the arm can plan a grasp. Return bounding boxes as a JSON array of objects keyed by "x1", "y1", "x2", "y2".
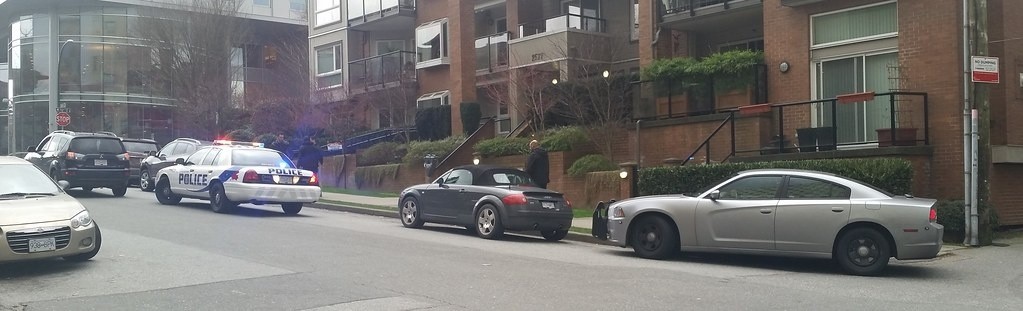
[
  {"x1": 139, "y1": 138, "x2": 213, "y2": 192},
  {"x1": 24, "y1": 130, "x2": 131, "y2": 198}
]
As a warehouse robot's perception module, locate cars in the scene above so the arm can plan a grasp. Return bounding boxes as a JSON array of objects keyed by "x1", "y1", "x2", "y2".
[
  {"x1": 120, "y1": 137, "x2": 161, "y2": 187},
  {"x1": 590, "y1": 168, "x2": 944, "y2": 276},
  {"x1": 398, "y1": 165, "x2": 574, "y2": 241},
  {"x1": 0, "y1": 155, "x2": 102, "y2": 264},
  {"x1": 154, "y1": 140, "x2": 322, "y2": 216}
]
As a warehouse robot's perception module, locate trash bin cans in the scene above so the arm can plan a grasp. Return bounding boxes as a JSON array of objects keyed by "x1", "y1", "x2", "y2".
[{"x1": 591, "y1": 198, "x2": 617, "y2": 240}]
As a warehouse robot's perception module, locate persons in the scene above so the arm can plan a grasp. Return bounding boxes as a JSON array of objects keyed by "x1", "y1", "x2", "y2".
[
  {"x1": 270, "y1": 133, "x2": 290, "y2": 155},
  {"x1": 525, "y1": 140, "x2": 550, "y2": 189},
  {"x1": 296, "y1": 136, "x2": 324, "y2": 197}
]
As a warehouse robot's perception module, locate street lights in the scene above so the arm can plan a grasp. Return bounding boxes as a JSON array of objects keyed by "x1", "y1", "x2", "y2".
[{"x1": 57, "y1": 39, "x2": 73, "y2": 130}]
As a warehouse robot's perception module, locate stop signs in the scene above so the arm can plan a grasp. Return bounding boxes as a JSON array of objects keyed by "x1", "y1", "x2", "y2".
[{"x1": 56, "y1": 112, "x2": 71, "y2": 127}]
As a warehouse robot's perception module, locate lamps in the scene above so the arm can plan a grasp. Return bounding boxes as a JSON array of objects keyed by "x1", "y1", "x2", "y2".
[{"x1": 779, "y1": 61, "x2": 789, "y2": 72}]
